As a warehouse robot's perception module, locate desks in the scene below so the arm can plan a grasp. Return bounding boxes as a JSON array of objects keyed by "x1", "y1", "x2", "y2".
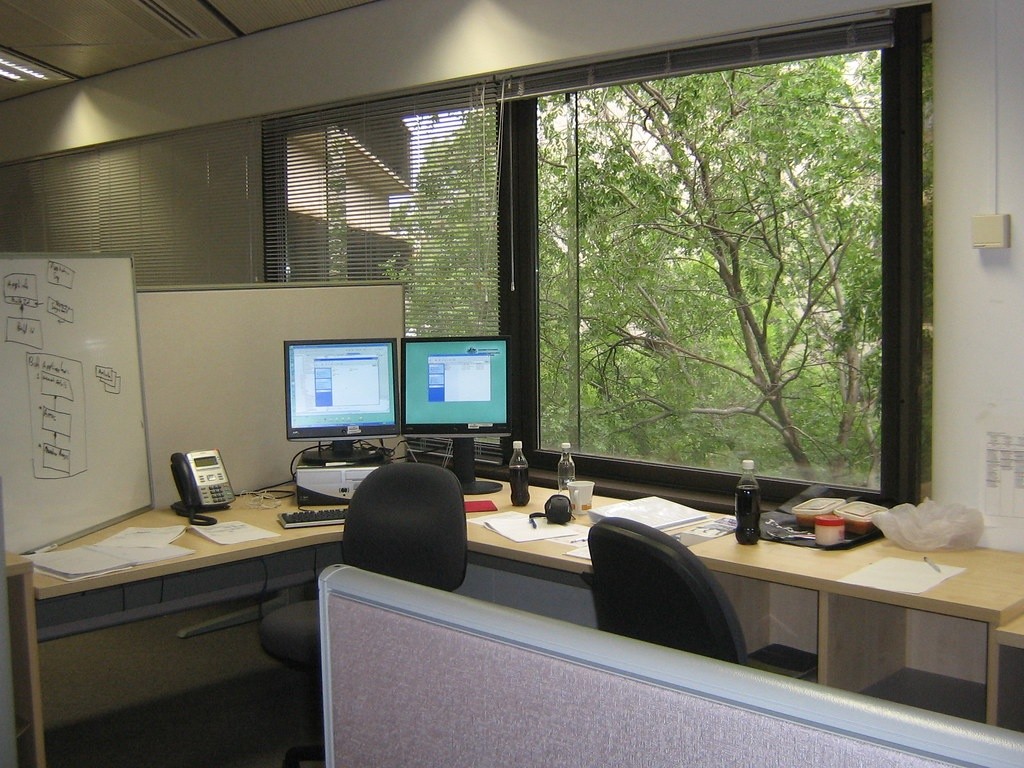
[{"x1": 0, "y1": 476, "x2": 1024, "y2": 768}]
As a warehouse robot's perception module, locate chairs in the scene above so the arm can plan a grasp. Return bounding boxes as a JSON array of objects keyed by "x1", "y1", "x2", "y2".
[
  {"x1": 259, "y1": 464, "x2": 468, "y2": 657},
  {"x1": 587, "y1": 517, "x2": 747, "y2": 665}
]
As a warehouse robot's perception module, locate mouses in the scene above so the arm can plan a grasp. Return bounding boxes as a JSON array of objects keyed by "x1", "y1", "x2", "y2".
[{"x1": 546, "y1": 495, "x2": 571, "y2": 524}]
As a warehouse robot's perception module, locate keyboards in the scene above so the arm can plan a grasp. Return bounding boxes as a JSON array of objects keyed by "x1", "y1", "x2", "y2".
[{"x1": 277, "y1": 509, "x2": 349, "y2": 529}]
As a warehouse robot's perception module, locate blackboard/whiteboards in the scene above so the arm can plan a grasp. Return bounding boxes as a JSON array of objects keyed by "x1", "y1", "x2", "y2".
[
  {"x1": 0, "y1": 249, "x2": 155, "y2": 560},
  {"x1": 132, "y1": 280, "x2": 408, "y2": 507}
]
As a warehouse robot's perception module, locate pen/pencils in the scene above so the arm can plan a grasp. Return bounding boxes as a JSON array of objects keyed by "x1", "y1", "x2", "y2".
[{"x1": 923, "y1": 556, "x2": 943, "y2": 573}]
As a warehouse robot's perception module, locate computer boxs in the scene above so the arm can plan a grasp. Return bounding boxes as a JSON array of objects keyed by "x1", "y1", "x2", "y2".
[{"x1": 296, "y1": 453, "x2": 387, "y2": 507}]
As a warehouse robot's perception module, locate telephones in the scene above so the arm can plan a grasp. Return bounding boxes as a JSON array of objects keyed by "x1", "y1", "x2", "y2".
[{"x1": 170, "y1": 448, "x2": 236, "y2": 513}]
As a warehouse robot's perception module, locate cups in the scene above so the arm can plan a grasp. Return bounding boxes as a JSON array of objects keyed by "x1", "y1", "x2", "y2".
[{"x1": 566, "y1": 480, "x2": 595, "y2": 515}]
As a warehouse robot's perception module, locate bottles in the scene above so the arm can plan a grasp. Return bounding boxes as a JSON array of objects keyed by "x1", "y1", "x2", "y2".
[
  {"x1": 558, "y1": 442, "x2": 575, "y2": 499},
  {"x1": 508, "y1": 441, "x2": 530, "y2": 507},
  {"x1": 734, "y1": 460, "x2": 762, "y2": 545}
]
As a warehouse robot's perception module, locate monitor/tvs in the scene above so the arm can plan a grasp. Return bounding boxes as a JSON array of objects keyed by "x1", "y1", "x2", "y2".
[
  {"x1": 401, "y1": 335, "x2": 512, "y2": 495},
  {"x1": 283, "y1": 338, "x2": 401, "y2": 463}
]
